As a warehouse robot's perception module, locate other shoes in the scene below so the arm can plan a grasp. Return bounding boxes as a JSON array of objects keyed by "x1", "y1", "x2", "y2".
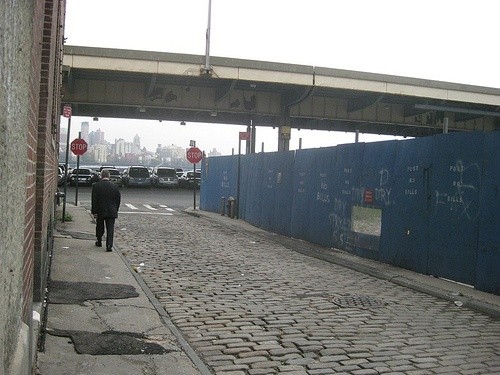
[
  {"x1": 106, "y1": 247, "x2": 113, "y2": 252},
  {"x1": 95, "y1": 241, "x2": 102, "y2": 247}
]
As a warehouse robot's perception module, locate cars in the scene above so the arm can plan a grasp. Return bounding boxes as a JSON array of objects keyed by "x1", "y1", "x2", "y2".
[{"x1": 59, "y1": 161, "x2": 201, "y2": 189}]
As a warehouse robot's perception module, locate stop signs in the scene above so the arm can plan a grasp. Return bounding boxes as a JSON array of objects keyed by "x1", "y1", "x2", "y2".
[
  {"x1": 71, "y1": 139, "x2": 88, "y2": 156},
  {"x1": 187, "y1": 148, "x2": 203, "y2": 163}
]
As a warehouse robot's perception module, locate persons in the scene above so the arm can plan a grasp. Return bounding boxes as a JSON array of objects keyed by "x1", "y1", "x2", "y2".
[{"x1": 92, "y1": 169, "x2": 121, "y2": 252}]
